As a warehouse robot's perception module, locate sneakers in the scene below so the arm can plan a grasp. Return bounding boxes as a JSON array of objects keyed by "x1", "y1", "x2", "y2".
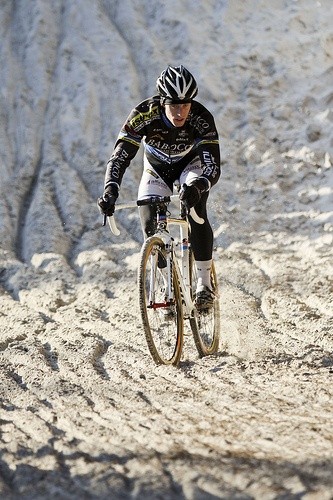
[
  {"x1": 161, "y1": 307, "x2": 171, "y2": 315},
  {"x1": 196, "y1": 282, "x2": 215, "y2": 308}
]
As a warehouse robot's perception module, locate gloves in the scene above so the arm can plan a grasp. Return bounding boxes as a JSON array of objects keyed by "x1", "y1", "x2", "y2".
[
  {"x1": 179, "y1": 178, "x2": 209, "y2": 208},
  {"x1": 97, "y1": 186, "x2": 119, "y2": 217}
]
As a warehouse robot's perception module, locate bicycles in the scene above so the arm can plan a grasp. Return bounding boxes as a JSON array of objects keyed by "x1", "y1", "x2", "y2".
[{"x1": 102, "y1": 182, "x2": 220, "y2": 368}]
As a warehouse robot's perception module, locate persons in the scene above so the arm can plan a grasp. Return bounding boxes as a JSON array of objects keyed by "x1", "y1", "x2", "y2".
[{"x1": 97, "y1": 66, "x2": 221, "y2": 310}]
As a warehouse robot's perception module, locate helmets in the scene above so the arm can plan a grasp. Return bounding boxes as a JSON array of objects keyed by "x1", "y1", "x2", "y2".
[{"x1": 156, "y1": 65, "x2": 199, "y2": 105}]
